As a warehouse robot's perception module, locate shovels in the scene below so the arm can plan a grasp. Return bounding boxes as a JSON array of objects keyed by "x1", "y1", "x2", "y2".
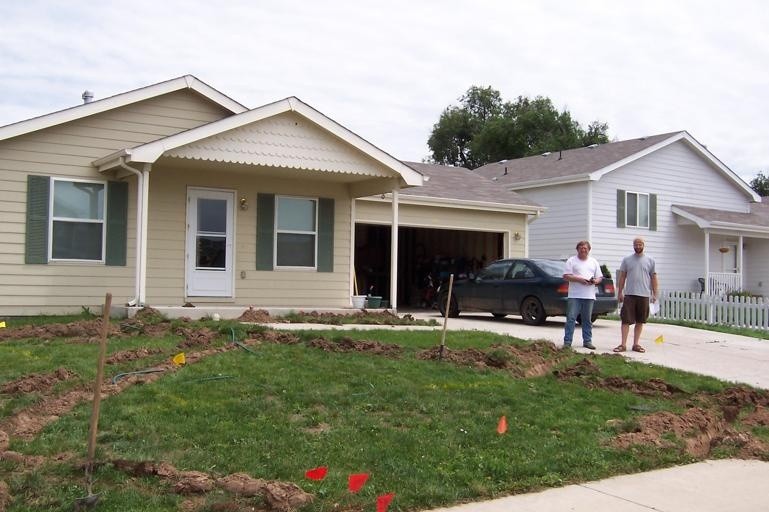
[{"x1": 73, "y1": 292, "x2": 113, "y2": 512}]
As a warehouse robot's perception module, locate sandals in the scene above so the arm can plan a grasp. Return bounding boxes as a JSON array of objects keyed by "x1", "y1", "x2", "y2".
[
  {"x1": 632, "y1": 344, "x2": 645, "y2": 353},
  {"x1": 613, "y1": 344, "x2": 626, "y2": 352}
]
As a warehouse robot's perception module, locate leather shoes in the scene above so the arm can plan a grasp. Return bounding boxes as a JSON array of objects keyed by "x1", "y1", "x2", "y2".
[{"x1": 583, "y1": 343, "x2": 595, "y2": 350}]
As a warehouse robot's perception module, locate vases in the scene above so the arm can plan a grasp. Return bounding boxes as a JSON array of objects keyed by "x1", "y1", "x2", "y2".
[
  {"x1": 380, "y1": 301, "x2": 389, "y2": 307},
  {"x1": 364, "y1": 300, "x2": 368, "y2": 307}
]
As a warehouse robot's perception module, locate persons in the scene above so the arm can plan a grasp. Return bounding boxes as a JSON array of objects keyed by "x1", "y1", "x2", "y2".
[
  {"x1": 560, "y1": 239, "x2": 605, "y2": 352},
  {"x1": 611, "y1": 236, "x2": 659, "y2": 353}
]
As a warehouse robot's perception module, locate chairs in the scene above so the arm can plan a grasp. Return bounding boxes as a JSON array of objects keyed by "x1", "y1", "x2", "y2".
[{"x1": 698, "y1": 277, "x2": 716, "y2": 299}]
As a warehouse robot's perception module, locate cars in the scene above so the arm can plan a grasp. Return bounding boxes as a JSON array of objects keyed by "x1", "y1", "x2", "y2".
[{"x1": 433, "y1": 257, "x2": 617, "y2": 325}]
